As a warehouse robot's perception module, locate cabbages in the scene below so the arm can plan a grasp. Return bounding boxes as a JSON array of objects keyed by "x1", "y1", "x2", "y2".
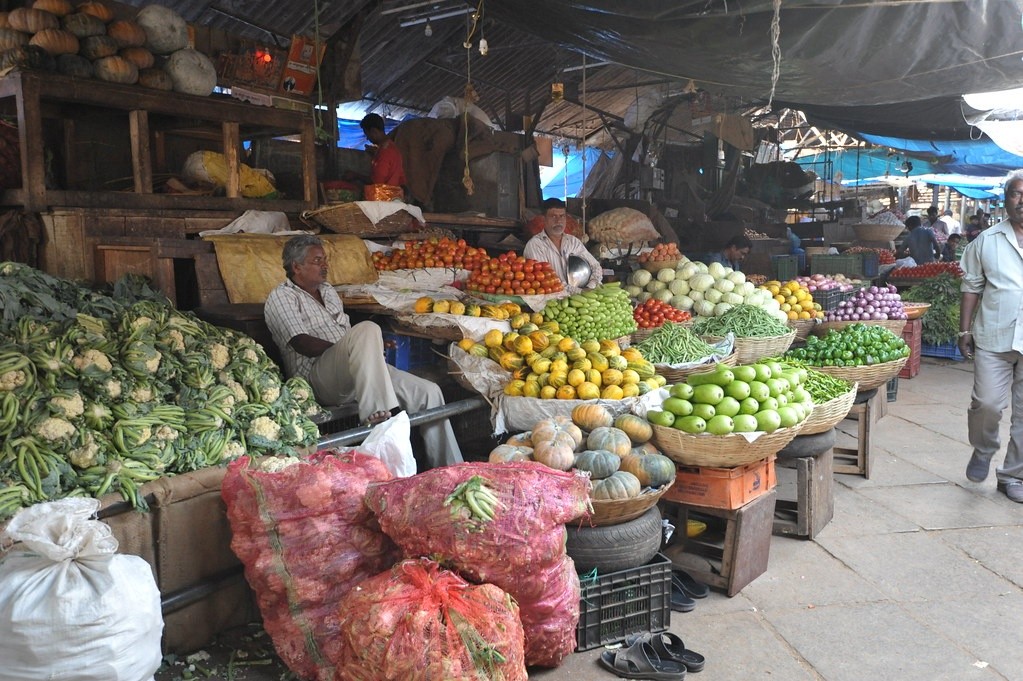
[
  {"x1": 694, "y1": 256, "x2": 788, "y2": 318},
  {"x1": 626, "y1": 257, "x2": 697, "y2": 310}
]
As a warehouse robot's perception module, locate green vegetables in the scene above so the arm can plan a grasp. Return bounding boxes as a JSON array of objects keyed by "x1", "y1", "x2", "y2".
[{"x1": 900, "y1": 270, "x2": 965, "y2": 346}]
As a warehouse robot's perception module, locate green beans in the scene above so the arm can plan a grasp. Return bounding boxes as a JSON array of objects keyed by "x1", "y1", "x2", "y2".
[
  {"x1": 693, "y1": 303, "x2": 791, "y2": 338},
  {"x1": 632, "y1": 321, "x2": 724, "y2": 365}
]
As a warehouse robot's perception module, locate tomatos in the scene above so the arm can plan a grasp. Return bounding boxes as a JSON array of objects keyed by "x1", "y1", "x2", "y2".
[
  {"x1": 371, "y1": 237, "x2": 562, "y2": 295},
  {"x1": 630, "y1": 299, "x2": 691, "y2": 328},
  {"x1": 843, "y1": 246, "x2": 896, "y2": 264},
  {"x1": 888, "y1": 261, "x2": 965, "y2": 277}
]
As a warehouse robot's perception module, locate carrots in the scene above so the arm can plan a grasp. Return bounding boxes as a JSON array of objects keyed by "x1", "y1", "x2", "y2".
[{"x1": 639, "y1": 242, "x2": 682, "y2": 262}]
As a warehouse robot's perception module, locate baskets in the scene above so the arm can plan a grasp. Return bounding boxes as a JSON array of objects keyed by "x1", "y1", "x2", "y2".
[
  {"x1": 852, "y1": 224, "x2": 906, "y2": 242},
  {"x1": 789, "y1": 319, "x2": 820, "y2": 343},
  {"x1": 612, "y1": 335, "x2": 631, "y2": 351},
  {"x1": 653, "y1": 336, "x2": 739, "y2": 384},
  {"x1": 903, "y1": 303, "x2": 932, "y2": 319},
  {"x1": 648, "y1": 402, "x2": 814, "y2": 467},
  {"x1": 566, "y1": 475, "x2": 676, "y2": 526},
  {"x1": 807, "y1": 354, "x2": 910, "y2": 393},
  {"x1": 630, "y1": 322, "x2": 696, "y2": 344},
  {"x1": 797, "y1": 381, "x2": 858, "y2": 435},
  {"x1": 640, "y1": 260, "x2": 678, "y2": 275},
  {"x1": 734, "y1": 328, "x2": 797, "y2": 365}
]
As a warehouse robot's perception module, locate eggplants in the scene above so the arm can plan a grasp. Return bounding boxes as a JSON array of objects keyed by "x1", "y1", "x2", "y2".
[{"x1": 795, "y1": 273, "x2": 909, "y2": 322}]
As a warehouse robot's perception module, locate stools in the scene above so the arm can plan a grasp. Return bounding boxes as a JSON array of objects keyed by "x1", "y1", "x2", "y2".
[
  {"x1": 833, "y1": 383, "x2": 887, "y2": 480},
  {"x1": 772, "y1": 446, "x2": 833, "y2": 539},
  {"x1": 656, "y1": 488, "x2": 776, "y2": 597}
]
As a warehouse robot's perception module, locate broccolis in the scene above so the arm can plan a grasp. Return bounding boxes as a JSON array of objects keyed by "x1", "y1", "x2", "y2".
[
  {"x1": 222, "y1": 451, "x2": 586, "y2": 681},
  {"x1": 0, "y1": 264, "x2": 319, "y2": 523}
]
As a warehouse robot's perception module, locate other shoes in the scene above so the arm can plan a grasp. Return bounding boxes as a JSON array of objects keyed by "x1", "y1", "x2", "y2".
[
  {"x1": 966, "y1": 449, "x2": 996, "y2": 482},
  {"x1": 997, "y1": 482, "x2": 1023, "y2": 503}
]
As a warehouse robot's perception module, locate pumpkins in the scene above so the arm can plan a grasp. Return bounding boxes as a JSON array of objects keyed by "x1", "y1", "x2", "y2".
[
  {"x1": 489, "y1": 403, "x2": 676, "y2": 498},
  {"x1": 0, "y1": 0, "x2": 217, "y2": 95},
  {"x1": 414, "y1": 295, "x2": 667, "y2": 402},
  {"x1": 760, "y1": 279, "x2": 825, "y2": 320}
]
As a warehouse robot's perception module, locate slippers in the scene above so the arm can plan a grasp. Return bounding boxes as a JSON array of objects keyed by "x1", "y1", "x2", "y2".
[
  {"x1": 669, "y1": 581, "x2": 696, "y2": 612},
  {"x1": 670, "y1": 569, "x2": 710, "y2": 598},
  {"x1": 600, "y1": 641, "x2": 687, "y2": 681},
  {"x1": 625, "y1": 631, "x2": 706, "y2": 673}
]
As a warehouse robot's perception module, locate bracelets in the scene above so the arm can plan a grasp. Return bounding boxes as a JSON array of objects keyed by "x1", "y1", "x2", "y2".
[{"x1": 958, "y1": 331, "x2": 972, "y2": 337}]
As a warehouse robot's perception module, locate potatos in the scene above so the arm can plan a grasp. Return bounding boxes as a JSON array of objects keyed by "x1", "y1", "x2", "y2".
[{"x1": 587, "y1": 206, "x2": 660, "y2": 242}]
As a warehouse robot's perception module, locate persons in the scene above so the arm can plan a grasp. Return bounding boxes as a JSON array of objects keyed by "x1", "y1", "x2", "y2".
[
  {"x1": 957, "y1": 173, "x2": 1023, "y2": 503},
  {"x1": 923, "y1": 206, "x2": 948, "y2": 235},
  {"x1": 894, "y1": 216, "x2": 940, "y2": 265},
  {"x1": 264, "y1": 234, "x2": 464, "y2": 468},
  {"x1": 349, "y1": 113, "x2": 406, "y2": 187},
  {"x1": 965, "y1": 209, "x2": 991, "y2": 242},
  {"x1": 709, "y1": 235, "x2": 752, "y2": 271},
  {"x1": 933, "y1": 234, "x2": 962, "y2": 262},
  {"x1": 523, "y1": 198, "x2": 603, "y2": 295}
]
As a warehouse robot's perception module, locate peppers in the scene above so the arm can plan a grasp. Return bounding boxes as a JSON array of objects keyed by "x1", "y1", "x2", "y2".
[
  {"x1": 786, "y1": 321, "x2": 912, "y2": 367},
  {"x1": 526, "y1": 215, "x2": 582, "y2": 237}
]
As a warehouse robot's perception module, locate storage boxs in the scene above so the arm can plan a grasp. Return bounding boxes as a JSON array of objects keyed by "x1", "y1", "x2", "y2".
[
  {"x1": 382, "y1": 333, "x2": 448, "y2": 371},
  {"x1": 574, "y1": 553, "x2": 672, "y2": 653},
  {"x1": 662, "y1": 452, "x2": 778, "y2": 510},
  {"x1": 886, "y1": 375, "x2": 898, "y2": 402},
  {"x1": 898, "y1": 319, "x2": 965, "y2": 379},
  {"x1": 777, "y1": 253, "x2": 887, "y2": 313}
]
